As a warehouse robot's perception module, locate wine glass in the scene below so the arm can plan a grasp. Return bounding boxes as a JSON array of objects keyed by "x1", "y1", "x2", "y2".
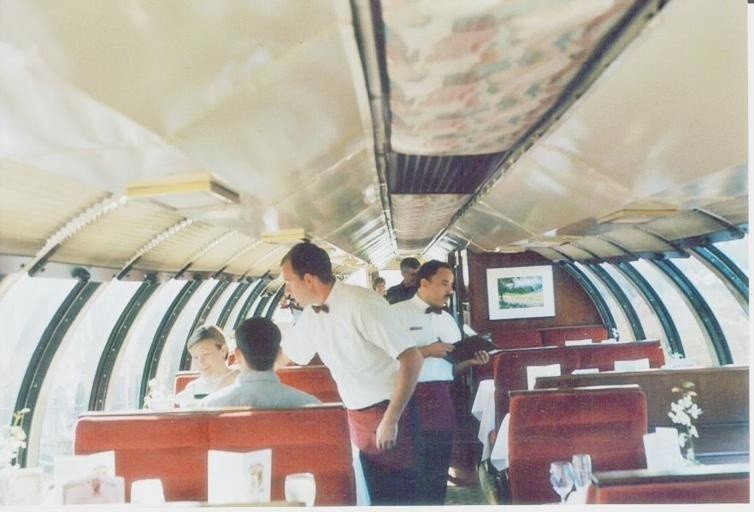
[
  {"x1": 284, "y1": 473, "x2": 316, "y2": 507},
  {"x1": 549, "y1": 461, "x2": 573, "y2": 504},
  {"x1": 572, "y1": 453, "x2": 592, "y2": 504}
]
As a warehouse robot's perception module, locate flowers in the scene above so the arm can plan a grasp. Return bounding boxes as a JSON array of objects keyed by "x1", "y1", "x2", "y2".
[
  {"x1": 668, "y1": 375, "x2": 704, "y2": 464},
  {"x1": 0, "y1": 408, "x2": 32, "y2": 472}
]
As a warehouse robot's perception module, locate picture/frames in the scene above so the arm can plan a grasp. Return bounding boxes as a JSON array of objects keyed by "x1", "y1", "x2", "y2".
[{"x1": 484, "y1": 261, "x2": 558, "y2": 322}]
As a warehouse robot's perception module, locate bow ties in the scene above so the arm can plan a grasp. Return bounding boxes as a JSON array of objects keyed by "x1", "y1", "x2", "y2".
[
  {"x1": 312, "y1": 305, "x2": 329, "y2": 314},
  {"x1": 425, "y1": 307, "x2": 442, "y2": 314}
]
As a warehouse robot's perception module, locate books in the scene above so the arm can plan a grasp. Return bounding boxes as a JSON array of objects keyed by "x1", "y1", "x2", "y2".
[{"x1": 443, "y1": 335, "x2": 501, "y2": 364}]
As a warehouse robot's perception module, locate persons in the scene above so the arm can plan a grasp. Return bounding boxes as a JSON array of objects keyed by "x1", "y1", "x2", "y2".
[
  {"x1": 271, "y1": 241, "x2": 455, "y2": 503},
  {"x1": 147, "y1": 323, "x2": 240, "y2": 410},
  {"x1": 391, "y1": 259, "x2": 490, "y2": 505},
  {"x1": 197, "y1": 318, "x2": 322, "y2": 408}
]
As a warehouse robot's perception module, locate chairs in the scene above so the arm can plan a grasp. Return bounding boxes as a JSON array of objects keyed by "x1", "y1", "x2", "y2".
[{"x1": 508, "y1": 383, "x2": 649, "y2": 504}]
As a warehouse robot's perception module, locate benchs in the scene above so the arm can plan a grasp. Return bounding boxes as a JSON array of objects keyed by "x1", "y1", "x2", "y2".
[
  {"x1": 487, "y1": 339, "x2": 667, "y2": 477},
  {"x1": 73, "y1": 402, "x2": 357, "y2": 506},
  {"x1": 585, "y1": 462, "x2": 750, "y2": 504},
  {"x1": 173, "y1": 364, "x2": 344, "y2": 403},
  {"x1": 475, "y1": 325, "x2": 608, "y2": 384}
]
